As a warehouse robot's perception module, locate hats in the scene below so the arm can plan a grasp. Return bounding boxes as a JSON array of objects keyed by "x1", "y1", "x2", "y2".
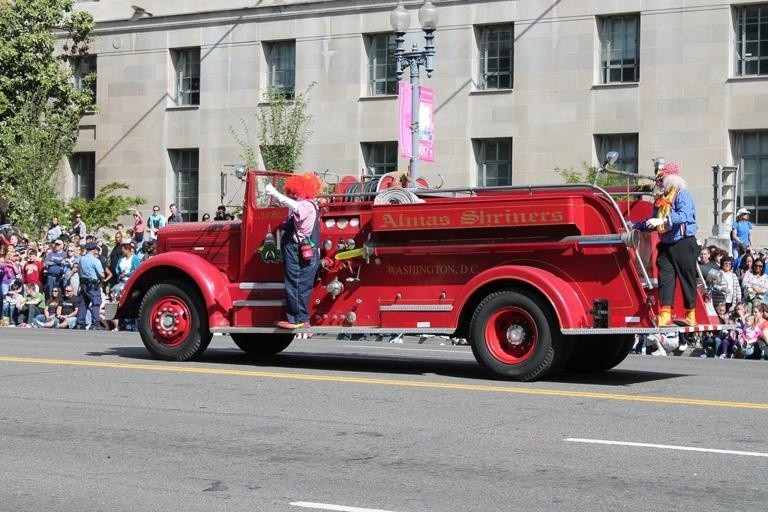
[
  {"x1": 736, "y1": 208, "x2": 751, "y2": 216},
  {"x1": 54, "y1": 239, "x2": 63, "y2": 245},
  {"x1": 86, "y1": 242, "x2": 97, "y2": 250}
]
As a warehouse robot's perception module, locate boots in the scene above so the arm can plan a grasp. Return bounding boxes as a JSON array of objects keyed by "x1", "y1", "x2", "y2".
[
  {"x1": 275, "y1": 320, "x2": 311, "y2": 330},
  {"x1": 675, "y1": 307, "x2": 699, "y2": 326},
  {"x1": 656, "y1": 305, "x2": 678, "y2": 327}
]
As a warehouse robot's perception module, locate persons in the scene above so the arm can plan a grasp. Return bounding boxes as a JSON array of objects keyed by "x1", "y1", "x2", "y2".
[
  {"x1": 642, "y1": 156, "x2": 700, "y2": 328},
  {"x1": 296, "y1": 333, "x2": 469, "y2": 346},
  {"x1": 235, "y1": 211, "x2": 243, "y2": 220},
  {"x1": 1, "y1": 202, "x2": 236, "y2": 331},
  {"x1": 266, "y1": 172, "x2": 321, "y2": 330},
  {"x1": 632, "y1": 208, "x2": 768, "y2": 359}
]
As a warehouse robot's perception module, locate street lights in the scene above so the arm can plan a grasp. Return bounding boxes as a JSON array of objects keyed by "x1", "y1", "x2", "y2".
[{"x1": 388, "y1": 0, "x2": 439, "y2": 190}]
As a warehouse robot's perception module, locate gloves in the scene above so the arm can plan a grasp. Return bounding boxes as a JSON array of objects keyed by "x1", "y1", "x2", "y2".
[{"x1": 645, "y1": 217, "x2": 667, "y2": 229}]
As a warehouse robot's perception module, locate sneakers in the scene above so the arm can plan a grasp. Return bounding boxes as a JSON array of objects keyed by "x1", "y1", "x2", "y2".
[
  {"x1": 0, "y1": 316, "x2": 140, "y2": 332},
  {"x1": 636, "y1": 340, "x2": 767, "y2": 361}
]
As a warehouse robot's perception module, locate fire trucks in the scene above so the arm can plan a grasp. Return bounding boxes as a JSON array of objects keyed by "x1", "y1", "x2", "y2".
[{"x1": 98, "y1": 149, "x2": 747, "y2": 385}]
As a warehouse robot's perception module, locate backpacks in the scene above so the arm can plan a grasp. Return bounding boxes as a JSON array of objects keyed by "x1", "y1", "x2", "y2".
[{"x1": 730, "y1": 221, "x2": 750, "y2": 241}]
